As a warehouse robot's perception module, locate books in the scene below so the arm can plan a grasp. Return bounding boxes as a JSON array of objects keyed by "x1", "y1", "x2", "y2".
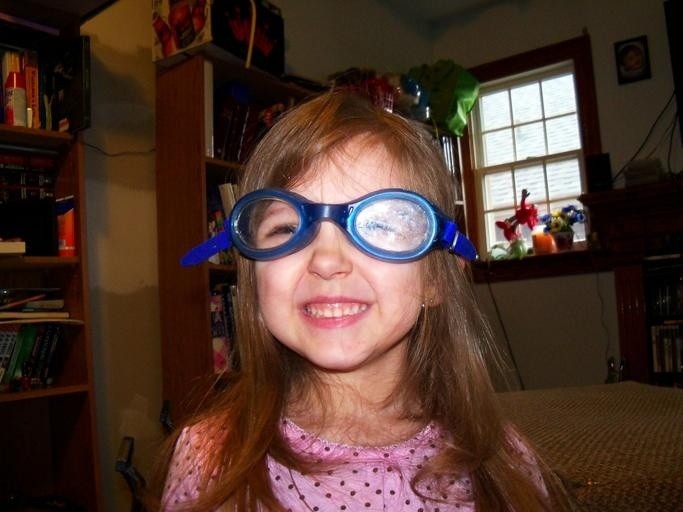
[
  {"x1": 0, "y1": 320, "x2": 81, "y2": 395},
  {"x1": 209, "y1": 282, "x2": 251, "y2": 376},
  {"x1": 208, "y1": 182, "x2": 242, "y2": 266},
  {"x1": 4, "y1": 48, "x2": 54, "y2": 130}
]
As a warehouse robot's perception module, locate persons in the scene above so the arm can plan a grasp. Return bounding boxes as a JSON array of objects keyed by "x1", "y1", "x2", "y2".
[{"x1": 158, "y1": 89, "x2": 553, "y2": 511}]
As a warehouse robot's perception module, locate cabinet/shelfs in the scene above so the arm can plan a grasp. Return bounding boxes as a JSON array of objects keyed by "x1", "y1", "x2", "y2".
[
  {"x1": 1, "y1": 12, "x2": 102, "y2": 512},
  {"x1": 154, "y1": 41, "x2": 320, "y2": 440},
  {"x1": 577, "y1": 176, "x2": 683, "y2": 387}
]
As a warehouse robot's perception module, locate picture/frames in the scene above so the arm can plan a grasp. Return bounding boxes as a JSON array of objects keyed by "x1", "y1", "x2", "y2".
[{"x1": 613, "y1": 34, "x2": 652, "y2": 87}]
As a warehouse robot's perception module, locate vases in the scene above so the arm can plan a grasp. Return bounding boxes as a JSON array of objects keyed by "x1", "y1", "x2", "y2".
[{"x1": 550, "y1": 231, "x2": 575, "y2": 253}]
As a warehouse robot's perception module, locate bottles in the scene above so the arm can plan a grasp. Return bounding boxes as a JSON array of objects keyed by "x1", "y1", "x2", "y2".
[
  {"x1": 152, "y1": 1, "x2": 206, "y2": 58},
  {"x1": 531, "y1": 225, "x2": 553, "y2": 256},
  {"x1": 506, "y1": 239, "x2": 528, "y2": 259},
  {"x1": 3, "y1": 71, "x2": 28, "y2": 126}
]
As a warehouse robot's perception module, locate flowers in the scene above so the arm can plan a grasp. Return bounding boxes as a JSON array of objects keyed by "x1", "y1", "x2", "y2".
[{"x1": 540, "y1": 205, "x2": 589, "y2": 235}]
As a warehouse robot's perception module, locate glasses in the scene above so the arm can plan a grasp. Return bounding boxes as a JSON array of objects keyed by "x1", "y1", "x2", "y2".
[{"x1": 177, "y1": 188, "x2": 482, "y2": 267}]
